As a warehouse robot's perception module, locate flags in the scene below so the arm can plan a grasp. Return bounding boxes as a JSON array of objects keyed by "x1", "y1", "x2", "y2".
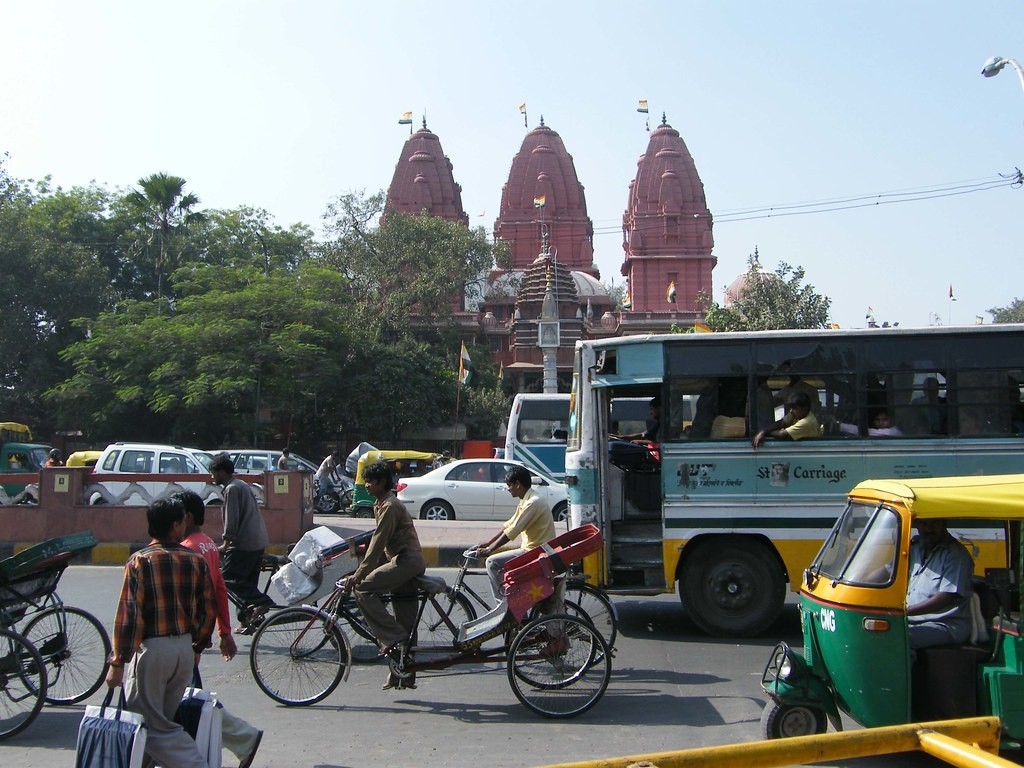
[
  {"x1": 534, "y1": 196, "x2": 545, "y2": 208},
  {"x1": 666, "y1": 283, "x2": 677, "y2": 303},
  {"x1": 519, "y1": 104, "x2": 526, "y2": 114},
  {"x1": 637, "y1": 99, "x2": 648, "y2": 113},
  {"x1": 622, "y1": 295, "x2": 631, "y2": 309},
  {"x1": 458, "y1": 345, "x2": 473, "y2": 384},
  {"x1": 398, "y1": 112, "x2": 412, "y2": 124}
]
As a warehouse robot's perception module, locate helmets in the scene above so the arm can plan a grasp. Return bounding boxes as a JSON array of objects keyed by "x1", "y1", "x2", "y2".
[{"x1": 50, "y1": 448, "x2": 62, "y2": 462}]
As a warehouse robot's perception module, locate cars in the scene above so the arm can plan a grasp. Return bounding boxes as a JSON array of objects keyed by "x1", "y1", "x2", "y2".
[
  {"x1": 88, "y1": 441, "x2": 356, "y2": 514},
  {"x1": 395, "y1": 458, "x2": 570, "y2": 524}
]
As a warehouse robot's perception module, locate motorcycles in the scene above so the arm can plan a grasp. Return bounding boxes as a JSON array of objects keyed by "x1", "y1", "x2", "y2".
[
  {"x1": 755, "y1": 479, "x2": 1024, "y2": 759},
  {"x1": 351, "y1": 450, "x2": 458, "y2": 518},
  {"x1": 66, "y1": 450, "x2": 117, "y2": 470}
]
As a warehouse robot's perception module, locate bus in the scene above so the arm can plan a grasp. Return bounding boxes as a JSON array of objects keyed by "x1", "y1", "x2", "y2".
[
  {"x1": 564, "y1": 323, "x2": 1024, "y2": 639},
  {"x1": 504, "y1": 391, "x2": 572, "y2": 485}
]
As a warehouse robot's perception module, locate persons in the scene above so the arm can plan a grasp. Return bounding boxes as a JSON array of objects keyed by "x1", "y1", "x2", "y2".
[
  {"x1": 611, "y1": 398, "x2": 660, "y2": 446},
  {"x1": 278, "y1": 447, "x2": 290, "y2": 469},
  {"x1": 472, "y1": 465, "x2": 569, "y2": 656},
  {"x1": 855, "y1": 517, "x2": 975, "y2": 672},
  {"x1": 520, "y1": 428, "x2": 568, "y2": 441},
  {"x1": 106, "y1": 489, "x2": 263, "y2": 768},
  {"x1": 335, "y1": 457, "x2": 348, "y2": 476},
  {"x1": 45, "y1": 447, "x2": 63, "y2": 468},
  {"x1": 414, "y1": 461, "x2": 428, "y2": 476},
  {"x1": 164, "y1": 458, "x2": 181, "y2": 473},
  {"x1": 686, "y1": 369, "x2": 1024, "y2": 447},
  {"x1": 346, "y1": 462, "x2": 426, "y2": 690},
  {"x1": 209, "y1": 454, "x2": 275, "y2": 634}
]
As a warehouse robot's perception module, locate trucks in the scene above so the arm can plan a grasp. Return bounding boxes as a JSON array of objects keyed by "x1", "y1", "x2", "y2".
[{"x1": 0, "y1": 422, "x2": 52, "y2": 504}]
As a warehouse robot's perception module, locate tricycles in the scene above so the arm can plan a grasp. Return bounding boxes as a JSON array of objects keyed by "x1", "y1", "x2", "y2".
[
  {"x1": 0, "y1": 530, "x2": 113, "y2": 742},
  {"x1": 248, "y1": 524, "x2": 613, "y2": 720}
]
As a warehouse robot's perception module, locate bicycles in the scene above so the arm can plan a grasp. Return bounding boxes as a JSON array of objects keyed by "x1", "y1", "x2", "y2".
[
  {"x1": 216, "y1": 549, "x2": 396, "y2": 663},
  {"x1": 313, "y1": 476, "x2": 354, "y2": 515},
  {"x1": 415, "y1": 542, "x2": 621, "y2": 670}
]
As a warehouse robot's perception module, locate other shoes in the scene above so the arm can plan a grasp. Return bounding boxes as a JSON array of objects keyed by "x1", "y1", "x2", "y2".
[
  {"x1": 251, "y1": 598, "x2": 273, "y2": 619},
  {"x1": 382, "y1": 677, "x2": 415, "y2": 690},
  {"x1": 234, "y1": 626, "x2": 256, "y2": 632},
  {"x1": 378, "y1": 636, "x2": 409, "y2": 657},
  {"x1": 539, "y1": 639, "x2": 568, "y2": 657},
  {"x1": 238, "y1": 730, "x2": 263, "y2": 768}
]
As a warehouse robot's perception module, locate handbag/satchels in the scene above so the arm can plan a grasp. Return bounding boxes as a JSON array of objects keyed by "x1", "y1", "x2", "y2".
[
  {"x1": 173, "y1": 663, "x2": 223, "y2": 768},
  {"x1": 74, "y1": 682, "x2": 148, "y2": 768}
]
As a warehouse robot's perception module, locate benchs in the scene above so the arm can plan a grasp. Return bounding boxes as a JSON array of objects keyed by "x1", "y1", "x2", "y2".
[{"x1": 918, "y1": 584, "x2": 1001, "y2": 665}]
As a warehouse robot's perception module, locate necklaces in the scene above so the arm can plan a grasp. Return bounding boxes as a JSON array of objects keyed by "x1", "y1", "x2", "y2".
[{"x1": 316, "y1": 450, "x2": 341, "y2": 500}]
